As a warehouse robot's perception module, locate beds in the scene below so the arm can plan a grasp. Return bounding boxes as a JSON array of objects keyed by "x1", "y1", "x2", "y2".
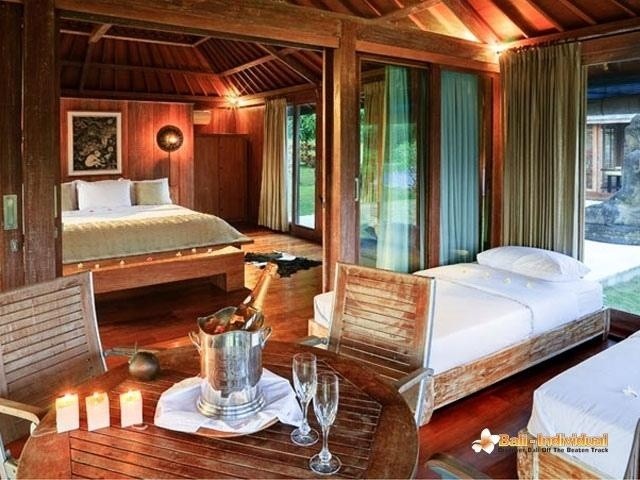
[
  {"x1": 515, "y1": 326, "x2": 640, "y2": 480},
  {"x1": 16, "y1": 339, "x2": 419, "y2": 480},
  {"x1": 306, "y1": 246, "x2": 610, "y2": 431},
  {"x1": 58, "y1": 176, "x2": 254, "y2": 295}
]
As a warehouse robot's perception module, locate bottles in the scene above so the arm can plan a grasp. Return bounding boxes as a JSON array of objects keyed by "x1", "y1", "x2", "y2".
[{"x1": 223, "y1": 263, "x2": 279, "y2": 332}]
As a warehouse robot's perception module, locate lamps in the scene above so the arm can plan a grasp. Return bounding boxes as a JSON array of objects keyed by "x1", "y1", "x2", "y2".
[{"x1": 156, "y1": 126, "x2": 183, "y2": 187}]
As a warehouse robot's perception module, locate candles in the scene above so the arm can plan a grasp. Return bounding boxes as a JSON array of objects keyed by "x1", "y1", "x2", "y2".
[
  {"x1": 83, "y1": 393, "x2": 111, "y2": 430},
  {"x1": 119, "y1": 390, "x2": 143, "y2": 427},
  {"x1": 56, "y1": 392, "x2": 81, "y2": 433}
]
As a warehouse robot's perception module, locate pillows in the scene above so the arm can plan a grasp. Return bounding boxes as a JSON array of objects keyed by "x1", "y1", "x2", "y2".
[
  {"x1": 75, "y1": 180, "x2": 130, "y2": 212},
  {"x1": 135, "y1": 178, "x2": 170, "y2": 204},
  {"x1": 477, "y1": 244, "x2": 592, "y2": 282}
]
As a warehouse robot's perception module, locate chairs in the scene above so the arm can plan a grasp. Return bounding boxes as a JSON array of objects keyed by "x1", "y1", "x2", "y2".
[
  {"x1": 300, "y1": 262, "x2": 438, "y2": 428},
  {"x1": 0, "y1": 270, "x2": 108, "y2": 480},
  {"x1": 422, "y1": 453, "x2": 496, "y2": 480}
]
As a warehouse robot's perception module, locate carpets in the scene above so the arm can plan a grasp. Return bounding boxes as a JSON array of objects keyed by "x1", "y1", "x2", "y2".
[{"x1": 246, "y1": 249, "x2": 323, "y2": 278}]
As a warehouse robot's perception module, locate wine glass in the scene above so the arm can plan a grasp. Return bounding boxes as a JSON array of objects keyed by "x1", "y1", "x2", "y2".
[{"x1": 291, "y1": 353, "x2": 341, "y2": 476}]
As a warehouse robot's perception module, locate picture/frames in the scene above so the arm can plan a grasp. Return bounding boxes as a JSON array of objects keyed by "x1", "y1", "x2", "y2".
[{"x1": 66, "y1": 110, "x2": 122, "y2": 176}]
